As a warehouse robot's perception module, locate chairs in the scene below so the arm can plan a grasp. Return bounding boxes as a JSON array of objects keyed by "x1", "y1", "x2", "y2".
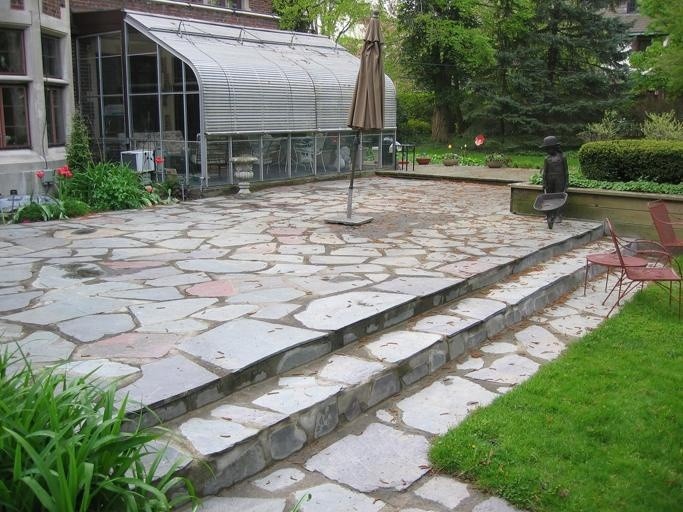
[
  {"x1": 188, "y1": 133, "x2": 229, "y2": 176},
  {"x1": 248, "y1": 132, "x2": 328, "y2": 176},
  {"x1": 602, "y1": 198, "x2": 681, "y2": 319}
]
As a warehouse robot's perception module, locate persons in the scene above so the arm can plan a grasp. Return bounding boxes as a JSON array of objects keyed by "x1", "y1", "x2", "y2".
[{"x1": 539, "y1": 135, "x2": 569, "y2": 229}]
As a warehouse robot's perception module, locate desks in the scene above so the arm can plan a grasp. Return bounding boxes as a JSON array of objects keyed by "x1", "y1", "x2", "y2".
[{"x1": 395, "y1": 143, "x2": 416, "y2": 171}]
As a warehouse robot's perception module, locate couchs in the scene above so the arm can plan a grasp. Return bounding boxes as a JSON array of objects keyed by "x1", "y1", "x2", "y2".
[{"x1": 133, "y1": 129, "x2": 187, "y2": 162}]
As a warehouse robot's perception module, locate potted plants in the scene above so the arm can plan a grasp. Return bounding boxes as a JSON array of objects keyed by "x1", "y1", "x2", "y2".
[
  {"x1": 485, "y1": 152, "x2": 507, "y2": 169},
  {"x1": 362, "y1": 144, "x2": 377, "y2": 177},
  {"x1": 417, "y1": 153, "x2": 431, "y2": 164},
  {"x1": 443, "y1": 153, "x2": 462, "y2": 166}
]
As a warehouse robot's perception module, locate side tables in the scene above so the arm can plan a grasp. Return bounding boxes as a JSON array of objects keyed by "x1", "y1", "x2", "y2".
[{"x1": 582, "y1": 253, "x2": 646, "y2": 307}]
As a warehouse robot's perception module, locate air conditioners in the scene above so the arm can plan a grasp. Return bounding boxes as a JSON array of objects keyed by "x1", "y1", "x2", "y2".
[{"x1": 120, "y1": 150, "x2": 155, "y2": 174}]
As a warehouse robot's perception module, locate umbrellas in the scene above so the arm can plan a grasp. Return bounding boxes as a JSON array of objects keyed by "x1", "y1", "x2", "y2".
[{"x1": 346, "y1": 10, "x2": 385, "y2": 218}]
